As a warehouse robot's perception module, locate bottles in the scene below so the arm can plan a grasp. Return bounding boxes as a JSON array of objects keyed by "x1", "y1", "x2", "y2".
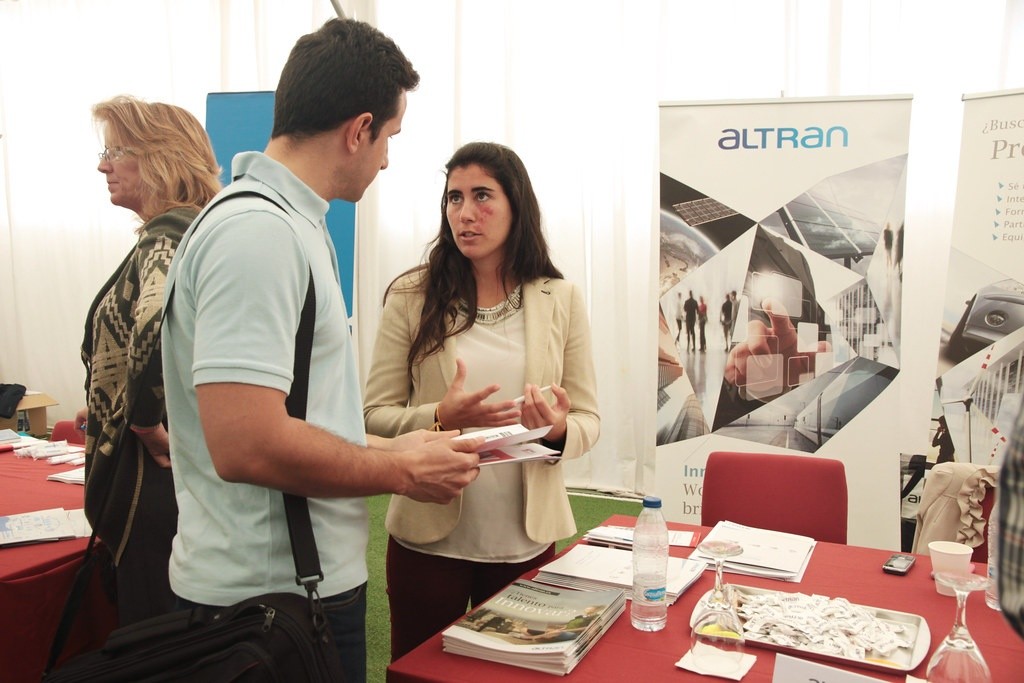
[{"x1": 629, "y1": 496, "x2": 668, "y2": 632}]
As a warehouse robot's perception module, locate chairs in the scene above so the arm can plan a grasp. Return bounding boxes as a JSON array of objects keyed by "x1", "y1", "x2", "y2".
[
  {"x1": 701, "y1": 453, "x2": 848, "y2": 542},
  {"x1": 913, "y1": 461, "x2": 999, "y2": 563},
  {"x1": 50, "y1": 418, "x2": 85, "y2": 446}
]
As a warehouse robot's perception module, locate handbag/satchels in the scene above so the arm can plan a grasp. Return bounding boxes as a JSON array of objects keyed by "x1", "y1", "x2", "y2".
[{"x1": 43, "y1": 593, "x2": 345, "y2": 682}]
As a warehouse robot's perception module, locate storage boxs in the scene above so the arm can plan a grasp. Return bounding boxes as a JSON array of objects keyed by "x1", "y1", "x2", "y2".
[{"x1": 0, "y1": 393, "x2": 61, "y2": 436}]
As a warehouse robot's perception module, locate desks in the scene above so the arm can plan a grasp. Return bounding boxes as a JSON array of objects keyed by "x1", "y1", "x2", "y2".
[
  {"x1": 0, "y1": 429, "x2": 119, "y2": 682},
  {"x1": 376, "y1": 513, "x2": 1024, "y2": 682}
]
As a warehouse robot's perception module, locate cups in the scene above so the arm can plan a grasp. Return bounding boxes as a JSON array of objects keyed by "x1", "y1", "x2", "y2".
[{"x1": 927, "y1": 540, "x2": 974, "y2": 596}]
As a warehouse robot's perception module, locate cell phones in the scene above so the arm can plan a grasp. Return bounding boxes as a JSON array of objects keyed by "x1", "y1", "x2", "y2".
[{"x1": 882, "y1": 553, "x2": 916, "y2": 576}]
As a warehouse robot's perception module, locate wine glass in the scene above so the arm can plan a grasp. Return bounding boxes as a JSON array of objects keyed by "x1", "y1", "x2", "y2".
[
  {"x1": 690, "y1": 541, "x2": 746, "y2": 665},
  {"x1": 925, "y1": 572, "x2": 994, "y2": 683}
]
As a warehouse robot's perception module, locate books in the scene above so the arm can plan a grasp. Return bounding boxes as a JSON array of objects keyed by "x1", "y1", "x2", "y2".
[{"x1": 441, "y1": 569, "x2": 627, "y2": 677}]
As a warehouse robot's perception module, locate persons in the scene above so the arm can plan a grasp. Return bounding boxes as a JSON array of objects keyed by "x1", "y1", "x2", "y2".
[
  {"x1": 166, "y1": 18, "x2": 483, "y2": 683},
  {"x1": 722, "y1": 298, "x2": 834, "y2": 406},
  {"x1": 510, "y1": 603, "x2": 605, "y2": 646},
  {"x1": 719, "y1": 290, "x2": 741, "y2": 353},
  {"x1": 883, "y1": 220, "x2": 904, "y2": 275},
  {"x1": 362, "y1": 141, "x2": 603, "y2": 683},
  {"x1": 673, "y1": 290, "x2": 710, "y2": 355},
  {"x1": 80, "y1": 101, "x2": 226, "y2": 624}
]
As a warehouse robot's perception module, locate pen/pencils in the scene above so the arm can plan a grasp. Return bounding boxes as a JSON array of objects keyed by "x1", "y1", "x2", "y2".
[
  {"x1": 512, "y1": 384, "x2": 552, "y2": 406},
  {"x1": 80, "y1": 421, "x2": 86, "y2": 430}
]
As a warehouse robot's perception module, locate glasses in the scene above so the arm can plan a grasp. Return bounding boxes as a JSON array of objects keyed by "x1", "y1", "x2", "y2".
[{"x1": 98, "y1": 146, "x2": 138, "y2": 164}]
